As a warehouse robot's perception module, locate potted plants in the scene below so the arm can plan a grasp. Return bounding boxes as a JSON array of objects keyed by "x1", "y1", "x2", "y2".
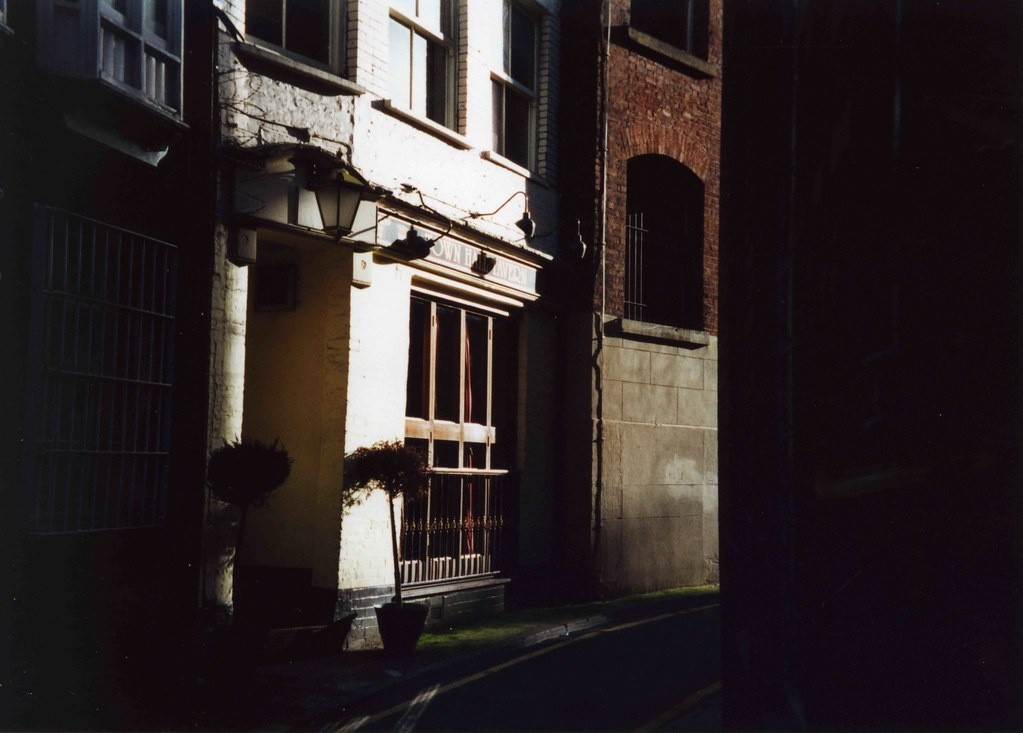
[
  {"x1": 208, "y1": 434, "x2": 293, "y2": 676},
  {"x1": 344, "y1": 438, "x2": 437, "y2": 654}
]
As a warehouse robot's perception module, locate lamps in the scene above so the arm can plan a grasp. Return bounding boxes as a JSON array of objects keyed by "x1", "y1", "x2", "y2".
[
  {"x1": 226, "y1": 141, "x2": 364, "y2": 241},
  {"x1": 533, "y1": 215, "x2": 587, "y2": 258},
  {"x1": 469, "y1": 191, "x2": 534, "y2": 240},
  {"x1": 399, "y1": 183, "x2": 453, "y2": 256}
]
